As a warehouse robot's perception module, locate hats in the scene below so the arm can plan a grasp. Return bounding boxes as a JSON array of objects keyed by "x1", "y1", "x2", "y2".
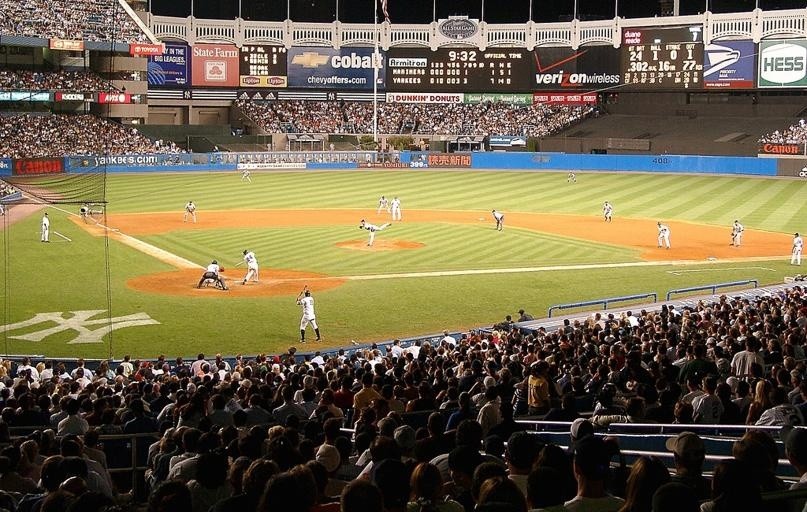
[
  {"x1": 570, "y1": 417, "x2": 594, "y2": 440},
  {"x1": 665, "y1": 431, "x2": 705, "y2": 460},
  {"x1": 779, "y1": 424, "x2": 806, "y2": 461}
]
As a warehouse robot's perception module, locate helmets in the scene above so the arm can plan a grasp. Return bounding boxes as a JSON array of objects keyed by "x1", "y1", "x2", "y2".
[{"x1": 212, "y1": 260, "x2": 217, "y2": 264}]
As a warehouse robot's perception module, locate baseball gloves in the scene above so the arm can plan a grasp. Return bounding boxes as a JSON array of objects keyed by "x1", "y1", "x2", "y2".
[
  {"x1": 358, "y1": 225, "x2": 362, "y2": 229},
  {"x1": 218, "y1": 266, "x2": 226, "y2": 272}
]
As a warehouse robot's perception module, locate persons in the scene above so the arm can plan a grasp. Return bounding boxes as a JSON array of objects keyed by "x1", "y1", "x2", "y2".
[
  {"x1": 241, "y1": 249, "x2": 258, "y2": 287},
  {"x1": 79, "y1": 204, "x2": 89, "y2": 223},
  {"x1": 729, "y1": 220, "x2": 744, "y2": 247},
  {"x1": 791, "y1": 233, "x2": 803, "y2": 266},
  {"x1": 196, "y1": 260, "x2": 227, "y2": 290},
  {"x1": 1, "y1": 1, "x2": 807, "y2": 204},
  {"x1": 42, "y1": 213, "x2": 50, "y2": 242},
  {"x1": 2, "y1": 276, "x2": 807, "y2": 512},
  {"x1": 655, "y1": 221, "x2": 670, "y2": 248},
  {"x1": 603, "y1": 201, "x2": 613, "y2": 222},
  {"x1": 492, "y1": 209, "x2": 505, "y2": 231},
  {"x1": 183, "y1": 200, "x2": 196, "y2": 223},
  {"x1": 359, "y1": 220, "x2": 391, "y2": 246},
  {"x1": 374, "y1": 194, "x2": 402, "y2": 221}
]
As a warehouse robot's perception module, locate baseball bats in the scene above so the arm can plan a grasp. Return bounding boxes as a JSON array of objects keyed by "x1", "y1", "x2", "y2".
[
  {"x1": 296, "y1": 285, "x2": 306, "y2": 300},
  {"x1": 234, "y1": 261, "x2": 246, "y2": 267}
]
